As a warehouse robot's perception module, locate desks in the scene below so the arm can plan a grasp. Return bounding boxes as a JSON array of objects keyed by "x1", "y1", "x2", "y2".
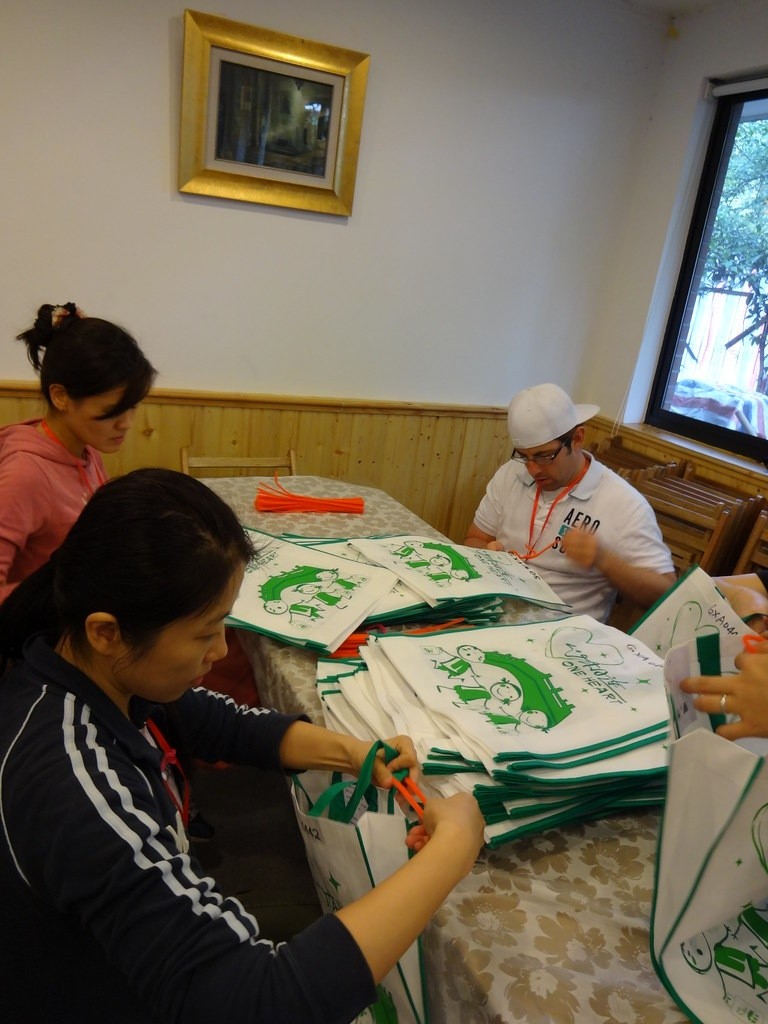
[{"x1": 194, "y1": 473, "x2": 699, "y2": 1024}]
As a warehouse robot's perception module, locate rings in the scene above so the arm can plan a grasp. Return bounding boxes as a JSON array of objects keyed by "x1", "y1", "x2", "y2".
[{"x1": 720, "y1": 695, "x2": 728, "y2": 714}]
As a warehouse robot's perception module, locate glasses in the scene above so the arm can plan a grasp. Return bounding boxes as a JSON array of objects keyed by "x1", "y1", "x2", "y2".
[{"x1": 511, "y1": 429, "x2": 575, "y2": 465}]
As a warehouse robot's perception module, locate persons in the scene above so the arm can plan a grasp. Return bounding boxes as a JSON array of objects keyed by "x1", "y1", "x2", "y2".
[
  {"x1": 1, "y1": 469, "x2": 486, "y2": 1023},
  {"x1": 0, "y1": 303, "x2": 155, "y2": 601},
  {"x1": 679, "y1": 599, "x2": 767, "y2": 739},
  {"x1": 462, "y1": 383, "x2": 678, "y2": 626}
]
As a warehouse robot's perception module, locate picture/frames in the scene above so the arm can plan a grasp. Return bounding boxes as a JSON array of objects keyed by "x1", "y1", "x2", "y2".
[{"x1": 175, "y1": 7, "x2": 374, "y2": 220}]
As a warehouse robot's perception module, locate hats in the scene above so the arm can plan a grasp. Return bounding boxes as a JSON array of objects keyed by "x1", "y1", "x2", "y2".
[{"x1": 507, "y1": 383, "x2": 601, "y2": 449}]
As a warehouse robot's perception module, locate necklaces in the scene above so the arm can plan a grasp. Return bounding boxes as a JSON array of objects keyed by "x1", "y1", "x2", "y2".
[
  {"x1": 147, "y1": 717, "x2": 192, "y2": 830},
  {"x1": 525, "y1": 458, "x2": 589, "y2": 562},
  {"x1": 41, "y1": 418, "x2": 103, "y2": 495}
]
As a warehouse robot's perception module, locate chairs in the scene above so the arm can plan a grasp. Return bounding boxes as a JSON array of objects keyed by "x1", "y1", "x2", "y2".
[
  {"x1": 179, "y1": 447, "x2": 297, "y2": 479},
  {"x1": 587, "y1": 434, "x2": 767, "y2": 636}
]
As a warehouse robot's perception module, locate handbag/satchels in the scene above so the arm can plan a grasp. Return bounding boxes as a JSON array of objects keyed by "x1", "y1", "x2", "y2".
[
  {"x1": 648, "y1": 633, "x2": 768, "y2": 1024},
  {"x1": 290, "y1": 738, "x2": 431, "y2": 1024},
  {"x1": 314, "y1": 612, "x2": 671, "y2": 848},
  {"x1": 222, "y1": 526, "x2": 573, "y2": 656},
  {"x1": 625, "y1": 561, "x2": 760, "y2": 659}
]
